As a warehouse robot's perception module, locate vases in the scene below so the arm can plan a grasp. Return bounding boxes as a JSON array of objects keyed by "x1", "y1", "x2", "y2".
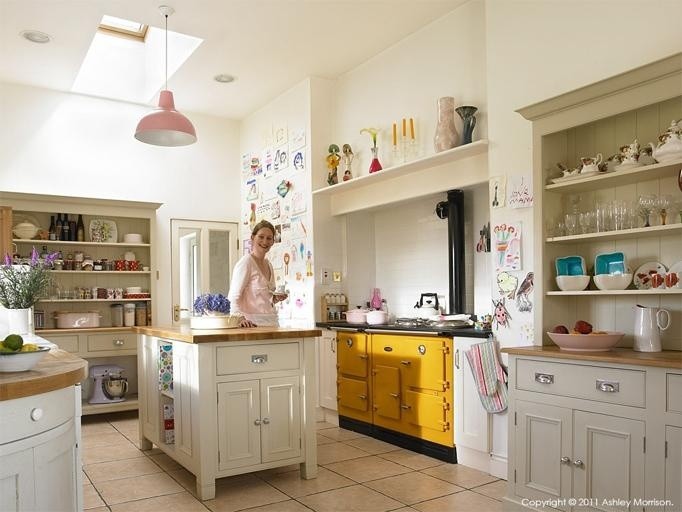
[
  {"x1": 203, "y1": 308, "x2": 224, "y2": 316},
  {"x1": 3, "y1": 305, "x2": 36, "y2": 338},
  {"x1": 367, "y1": 146, "x2": 382, "y2": 176}
]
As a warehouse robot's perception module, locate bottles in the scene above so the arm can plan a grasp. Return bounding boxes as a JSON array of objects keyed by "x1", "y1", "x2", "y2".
[
  {"x1": 325, "y1": 293, "x2": 345, "y2": 304},
  {"x1": 328, "y1": 311, "x2": 346, "y2": 320},
  {"x1": 380, "y1": 299, "x2": 388, "y2": 324},
  {"x1": 39, "y1": 245, "x2": 91, "y2": 270},
  {"x1": 110, "y1": 302, "x2": 147, "y2": 327},
  {"x1": 371, "y1": 287, "x2": 381, "y2": 310},
  {"x1": 48, "y1": 212, "x2": 85, "y2": 242},
  {"x1": 99, "y1": 258, "x2": 108, "y2": 269},
  {"x1": 34, "y1": 308, "x2": 45, "y2": 330}
]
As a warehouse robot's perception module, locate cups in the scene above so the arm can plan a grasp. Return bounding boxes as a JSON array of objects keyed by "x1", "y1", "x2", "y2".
[
  {"x1": 275, "y1": 283, "x2": 288, "y2": 294},
  {"x1": 113, "y1": 259, "x2": 143, "y2": 272},
  {"x1": 546, "y1": 197, "x2": 636, "y2": 238},
  {"x1": 37, "y1": 286, "x2": 75, "y2": 299},
  {"x1": 76, "y1": 287, "x2": 123, "y2": 301},
  {"x1": 475, "y1": 315, "x2": 492, "y2": 331}
]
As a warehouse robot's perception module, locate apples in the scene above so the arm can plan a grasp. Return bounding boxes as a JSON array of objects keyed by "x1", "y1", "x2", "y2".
[
  {"x1": 552, "y1": 326, "x2": 569, "y2": 334},
  {"x1": 575, "y1": 321, "x2": 592, "y2": 334}
]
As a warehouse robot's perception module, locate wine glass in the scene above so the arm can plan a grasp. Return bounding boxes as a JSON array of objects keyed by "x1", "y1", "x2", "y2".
[
  {"x1": 639, "y1": 192, "x2": 671, "y2": 226},
  {"x1": 455, "y1": 105, "x2": 478, "y2": 145}
]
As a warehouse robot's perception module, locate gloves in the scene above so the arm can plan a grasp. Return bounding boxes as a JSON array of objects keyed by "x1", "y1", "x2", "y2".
[{"x1": 465, "y1": 340, "x2": 509, "y2": 415}]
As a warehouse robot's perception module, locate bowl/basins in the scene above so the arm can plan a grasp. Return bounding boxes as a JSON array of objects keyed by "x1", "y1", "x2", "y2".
[
  {"x1": 555, "y1": 275, "x2": 591, "y2": 292},
  {"x1": 123, "y1": 233, "x2": 143, "y2": 244},
  {"x1": 555, "y1": 255, "x2": 588, "y2": 276},
  {"x1": 191, "y1": 314, "x2": 241, "y2": 329},
  {"x1": 51, "y1": 310, "x2": 102, "y2": 329},
  {"x1": 126, "y1": 287, "x2": 142, "y2": 293},
  {"x1": 11, "y1": 220, "x2": 42, "y2": 240},
  {"x1": 1, "y1": 346, "x2": 51, "y2": 374},
  {"x1": 142, "y1": 266, "x2": 149, "y2": 271},
  {"x1": 546, "y1": 332, "x2": 626, "y2": 354},
  {"x1": 594, "y1": 251, "x2": 629, "y2": 274},
  {"x1": 592, "y1": 274, "x2": 632, "y2": 292}
]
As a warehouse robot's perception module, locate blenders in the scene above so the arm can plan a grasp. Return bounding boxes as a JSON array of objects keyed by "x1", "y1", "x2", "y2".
[{"x1": 87, "y1": 365, "x2": 128, "y2": 405}]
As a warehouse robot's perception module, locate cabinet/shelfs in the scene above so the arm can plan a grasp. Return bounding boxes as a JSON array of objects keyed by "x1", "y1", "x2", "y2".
[
  {"x1": 317, "y1": 327, "x2": 338, "y2": 413},
  {"x1": 535, "y1": 67, "x2": 682, "y2": 353},
  {"x1": 135, "y1": 333, "x2": 328, "y2": 501},
  {"x1": 454, "y1": 335, "x2": 508, "y2": 481},
  {"x1": 0, "y1": 383, "x2": 84, "y2": 512},
  {"x1": 499, "y1": 353, "x2": 682, "y2": 512},
  {"x1": 37, "y1": 331, "x2": 141, "y2": 420},
  {"x1": 0, "y1": 191, "x2": 164, "y2": 334}
]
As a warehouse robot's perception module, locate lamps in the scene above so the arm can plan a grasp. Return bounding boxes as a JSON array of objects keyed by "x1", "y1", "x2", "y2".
[{"x1": 133, "y1": 4, "x2": 199, "y2": 147}]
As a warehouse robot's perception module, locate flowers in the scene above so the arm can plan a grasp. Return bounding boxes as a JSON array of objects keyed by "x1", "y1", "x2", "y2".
[
  {"x1": 359, "y1": 127, "x2": 379, "y2": 152},
  {"x1": 193, "y1": 293, "x2": 231, "y2": 314},
  {"x1": 0, "y1": 245, "x2": 64, "y2": 310}
]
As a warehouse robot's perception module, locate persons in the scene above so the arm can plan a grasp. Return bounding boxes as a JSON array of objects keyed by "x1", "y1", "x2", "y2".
[{"x1": 229, "y1": 219, "x2": 289, "y2": 328}]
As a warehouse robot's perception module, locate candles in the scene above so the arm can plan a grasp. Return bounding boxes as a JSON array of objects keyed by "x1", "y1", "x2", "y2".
[
  {"x1": 392, "y1": 122, "x2": 397, "y2": 145},
  {"x1": 402, "y1": 117, "x2": 407, "y2": 136},
  {"x1": 410, "y1": 118, "x2": 415, "y2": 139}
]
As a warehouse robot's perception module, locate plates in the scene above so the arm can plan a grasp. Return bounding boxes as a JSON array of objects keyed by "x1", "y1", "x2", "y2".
[
  {"x1": 123, "y1": 251, "x2": 135, "y2": 261},
  {"x1": 632, "y1": 260, "x2": 682, "y2": 290},
  {"x1": 550, "y1": 163, "x2": 640, "y2": 184},
  {"x1": 89, "y1": 219, "x2": 117, "y2": 244}
]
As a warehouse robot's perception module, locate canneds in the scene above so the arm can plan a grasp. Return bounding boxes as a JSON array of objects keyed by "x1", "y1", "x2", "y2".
[
  {"x1": 64, "y1": 251, "x2": 103, "y2": 271},
  {"x1": 329, "y1": 311, "x2": 346, "y2": 320}
]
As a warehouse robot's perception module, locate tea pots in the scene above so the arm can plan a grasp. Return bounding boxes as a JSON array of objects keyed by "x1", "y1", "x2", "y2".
[
  {"x1": 631, "y1": 301, "x2": 673, "y2": 353},
  {"x1": 647, "y1": 118, "x2": 682, "y2": 164},
  {"x1": 579, "y1": 152, "x2": 603, "y2": 173},
  {"x1": 413, "y1": 292, "x2": 442, "y2": 318}
]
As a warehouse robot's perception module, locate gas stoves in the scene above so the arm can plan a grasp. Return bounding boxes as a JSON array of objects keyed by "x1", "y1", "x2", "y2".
[{"x1": 385, "y1": 317, "x2": 470, "y2": 331}]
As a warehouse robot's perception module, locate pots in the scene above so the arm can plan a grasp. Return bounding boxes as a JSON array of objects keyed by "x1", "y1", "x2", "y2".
[
  {"x1": 342, "y1": 305, "x2": 369, "y2": 325},
  {"x1": 363, "y1": 307, "x2": 386, "y2": 326}
]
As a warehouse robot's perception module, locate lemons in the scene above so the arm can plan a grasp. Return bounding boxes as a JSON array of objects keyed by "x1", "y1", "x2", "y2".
[{"x1": 0, "y1": 334, "x2": 38, "y2": 355}]
